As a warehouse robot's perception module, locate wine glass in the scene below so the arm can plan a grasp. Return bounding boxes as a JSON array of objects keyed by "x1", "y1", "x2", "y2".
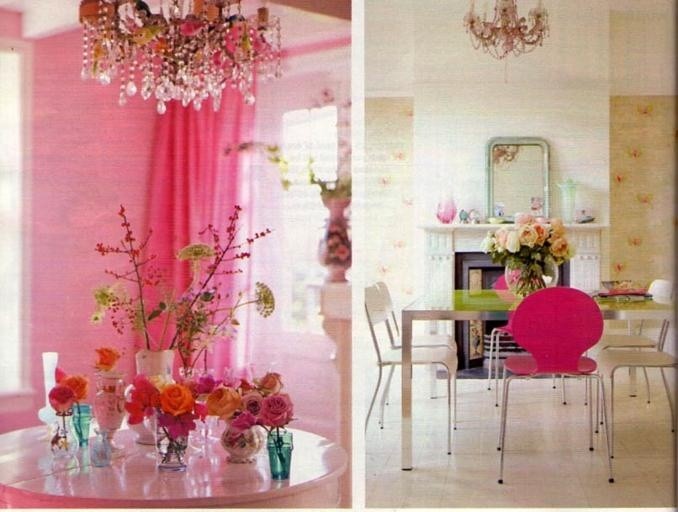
[{"x1": 95, "y1": 372, "x2": 128, "y2": 460}]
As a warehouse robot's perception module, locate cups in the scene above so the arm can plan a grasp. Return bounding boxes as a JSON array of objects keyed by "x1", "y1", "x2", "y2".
[
  {"x1": 154, "y1": 412, "x2": 187, "y2": 471},
  {"x1": 72, "y1": 404, "x2": 92, "y2": 449}
]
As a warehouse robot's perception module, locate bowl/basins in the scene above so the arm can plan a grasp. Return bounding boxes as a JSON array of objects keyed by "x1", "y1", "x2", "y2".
[{"x1": 601, "y1": 279, "x2": 631, "y2": 292}]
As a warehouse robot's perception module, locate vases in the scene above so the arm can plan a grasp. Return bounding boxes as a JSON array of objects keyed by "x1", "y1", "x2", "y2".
[
  {"x1": 152, "y1": 419, "x2": 191, "y2": 470},
  {"x1": 93, "y1": 378, "x2": 126, "y2": 434},
  {"x1": 52, "y1": 429, "x2": 73, "y2": 460},
  {"x1": 135, "y1": 348, "x2": 174, "y2": 421},
  {"x1": 505, "y1": 256, "x2": 558, "y2": 295},
  {"x1": 220, "y1": 422, "x2": 262, "y2": 463},
  {"x1": 318, "y1": 200, "x2": 351, "y2": 283},
  {"x1": 89, "y1": 427, "x2": 112, "y2": 467},
  {"x1": 71, "y1": 405, "x2": 91, "y2": 444}
]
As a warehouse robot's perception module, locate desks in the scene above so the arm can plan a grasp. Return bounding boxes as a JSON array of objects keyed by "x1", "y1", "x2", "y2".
[{"x1": 0, "y1": 420, "x2": 347, "y2": 508}]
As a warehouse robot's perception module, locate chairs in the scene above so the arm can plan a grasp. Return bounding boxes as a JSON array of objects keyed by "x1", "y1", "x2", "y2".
[
  {"x1": 363, "y1": 286, "x2": 457, "y2": 454},
  {"x1": 497, "y1": 288, "x2": 614, "y2": 484},
  {"x1": 598, "y1": 309, "x2": 678, "y2": 458},
  {"x1": 377, "y1": 281, "x2": 459, "y2": 424},
  {"x1": 488, "y1": 274, "x2": 567, "y2": 408},
  {"x1": 598, "y1": 280, "x2": 676, "y2": 425}
]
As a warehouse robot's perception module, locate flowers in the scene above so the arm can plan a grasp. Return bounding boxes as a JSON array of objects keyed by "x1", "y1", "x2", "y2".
[
  {"x1": 92, "y1": 350, "x2": 124, "y2": 433},
  {"x1": 139, "y1": 377, "x2": 216, "y2": 442},
  {"x1": 312, "y1": 174, "x2": 354, "y2": 283},
  {"x1": 49, "y1": 387, "x2": 73, "y2": 449},
  {"x1": 261, "y1": 392, "x2": 293, "y2": 469},
  {"x1": 484, "y1": 216, "x2": 573, "y2": 296},
  {"x1": 210, "y1": 373, "x2": 293, "y2": 430},
  {"x1": 401, "y1": 288, "x2": 676, "y2": 471}
]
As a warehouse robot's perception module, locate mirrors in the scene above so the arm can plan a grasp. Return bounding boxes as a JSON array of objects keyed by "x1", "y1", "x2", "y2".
[{"x1": 486, "y1": 137, "x2": 550, "y2": 222}]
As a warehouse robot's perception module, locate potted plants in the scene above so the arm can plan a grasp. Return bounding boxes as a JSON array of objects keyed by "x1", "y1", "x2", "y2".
[
  {"x1": 93, "y1": 202, "x2": 274, "y2": 444},
  {"x1": 59, "y1": 378, "x2": 99, "y2": 427}
]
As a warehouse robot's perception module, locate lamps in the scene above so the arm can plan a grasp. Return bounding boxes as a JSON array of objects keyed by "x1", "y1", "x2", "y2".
[
  {"x1": 78, "y1": 1, "x2": 282, "y2": 114},
  {"x1": 463, "y1": 1, "x2": 549, "y2": 59}
]
